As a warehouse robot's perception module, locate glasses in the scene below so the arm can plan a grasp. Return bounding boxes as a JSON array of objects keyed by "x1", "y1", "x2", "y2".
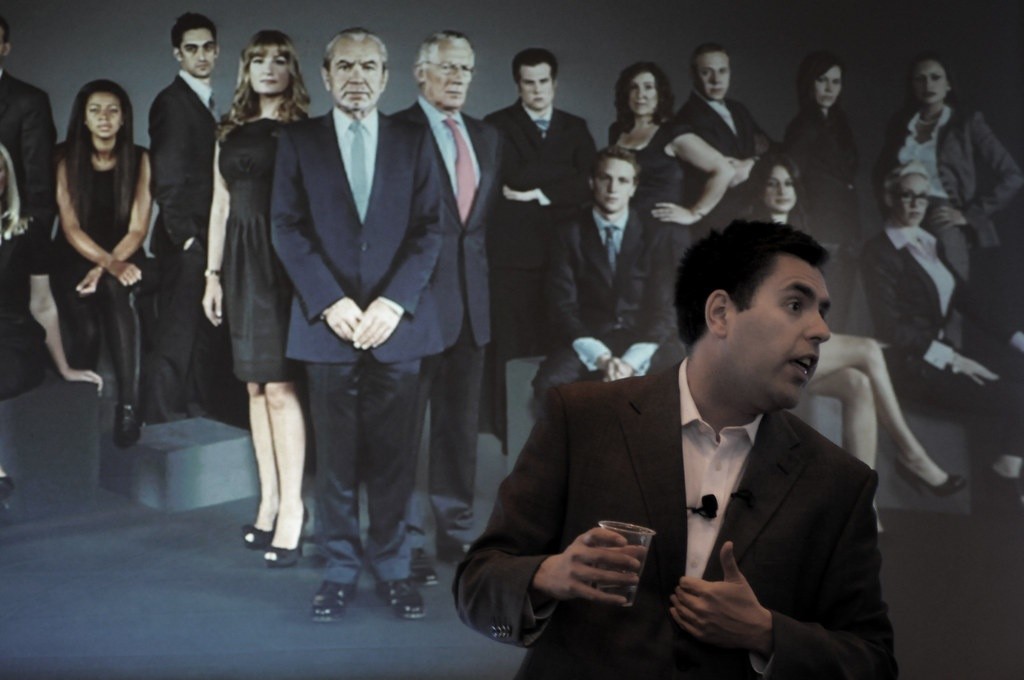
[
  {"x1": 419, "y1": 60, "x2": 475, "y2": 79},
  {"x1": 887, "y1": 189, "x2": 928, "y2": 205}
]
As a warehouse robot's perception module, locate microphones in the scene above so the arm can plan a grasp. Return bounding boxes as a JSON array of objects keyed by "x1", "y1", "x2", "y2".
[{"x1": 702, "y1": 495, "x2": 718, "y2": 518}]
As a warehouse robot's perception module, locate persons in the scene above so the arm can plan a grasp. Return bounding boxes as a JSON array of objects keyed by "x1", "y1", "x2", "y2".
[
  {"x1": 608, "y1": 61, "x2": 736, "y2": 282},
  {"x1": 202, "y1": 30, "x2": 312, "y2": 566},
  {"x1": 451, "y1": 219, "x2": 899, "y2": 680},
  {"x1": 0, "y1": 17, "x2": 103, "y2": 495},
  {"x1": 388, "y1": 31, "x2": 502, "y2": 588},
  {"x1": 270, "y1": 27, "x2": 445, "y2": 622},
  {"x1": 54, "y1": 80, "x2": 155, "y2": 448},
  {"x1": 481, "y1": 48, "x2": 597, "y2": 456},
  {"x1": 531, "y1": 146, "x2": 686, "y2": 419},
  {"x1": 673, "y1": 43, "x2": 1024, "y2": 548},
  {"x1": 148, "y1": 12, "x2": 239, "y2": 429}
]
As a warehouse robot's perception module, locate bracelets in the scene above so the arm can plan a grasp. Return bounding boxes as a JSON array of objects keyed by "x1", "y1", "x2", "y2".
[
  {"x1": 204, "y1": 270, "x2": 221, "y2": 277},
  {"x1": 692, "y1": 206, "x2": 706, "y2": 217}
]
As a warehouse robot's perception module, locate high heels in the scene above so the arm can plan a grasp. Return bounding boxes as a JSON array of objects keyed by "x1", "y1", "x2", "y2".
[
  {"x1": 266, "y1": 505, "x2": 308, "y2": 567},
  {"x1": 243, "y1": 522, "x2": 275, "y2": 547},
  {"x1": 895, "y1": 457, "x2": 968, "y2": 499}
]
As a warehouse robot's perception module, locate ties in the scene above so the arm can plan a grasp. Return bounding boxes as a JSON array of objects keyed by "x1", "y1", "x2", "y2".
[
  {"x1": 604, "y1": 226, "x2": 619, "y2": 277},
  {"x1": 442, "y1": 119, "x2": 476, "y2": 225},
  {"x1": 535, "y1": 118, "x2": 549, "y2": 140},
  {"x1": 348, "y1": 122, "x2": 367, "y2": 224}
]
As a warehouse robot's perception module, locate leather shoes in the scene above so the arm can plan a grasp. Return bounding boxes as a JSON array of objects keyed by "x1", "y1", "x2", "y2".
[
  {"x1": 435, "y1": 533, "x2": 474, "y2": 563},
  {"x1": 312, "y1": 580, "x2": 357, "y2": 615},
  {"x1": 373, "y1": 573, "x2": 425, "y2": 618},
  {"x1": 114, "y1": 401, "x2": 142, "y2": 448},
  {"x1": 410, "y1": 548, "x2": 438, "y2": 586}
]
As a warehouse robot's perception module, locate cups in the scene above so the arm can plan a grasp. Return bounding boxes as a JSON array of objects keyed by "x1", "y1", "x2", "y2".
[{"x1": 596, "y1": 520, "x2": 656, "y2": 607}]
